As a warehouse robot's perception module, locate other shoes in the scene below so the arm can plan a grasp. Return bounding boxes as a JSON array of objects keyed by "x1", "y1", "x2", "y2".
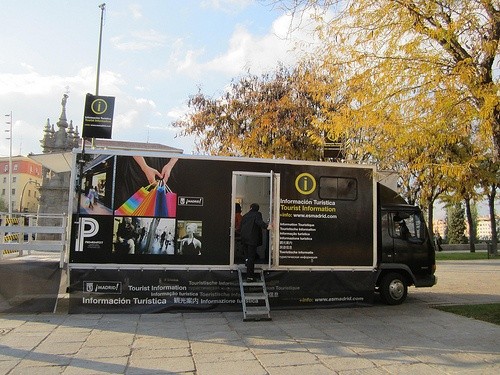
[{"x1": 246, "y1": 278, "x2": 255, "y2": 283}]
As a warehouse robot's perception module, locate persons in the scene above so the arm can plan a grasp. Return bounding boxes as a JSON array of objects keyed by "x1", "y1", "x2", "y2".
[
  {"x1": 135, "y1": 225, "x2": 146, "y2": 244},
  {"x1": 94, "y1": 186, "x2": 99, "y2": 206},
  {"x1": 117, "y1": 217, "x2": 135, "y2": 254},
  {"x1": 235, "y1": 203, "x2": 243, "y2": 238},
  {"x1": 131, "y1": 156, "x2": 179, "y2": 187},
  {"x1": 240, "y1": 203, "x2": 269, "y2": 281},
  {"x1": 88, "y1": 186, "x2": 95, "y2": 208},
  {"x1": 156, "y1": 231, "x2": 172, "y2": 251},
  {"x1": 180, "y1": 223, "x2": 201, "y2": 256},
  {"x1": 433, "y1": 233, "x2": 443, "y2": 251},
  {"x1": 98, "y1": 179, "x2": 106, "y2": 196}
]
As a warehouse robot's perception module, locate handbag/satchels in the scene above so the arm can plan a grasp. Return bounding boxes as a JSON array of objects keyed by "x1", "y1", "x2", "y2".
[{"x1": 115, "y1": 178, "x2": 177, "y2": 217}]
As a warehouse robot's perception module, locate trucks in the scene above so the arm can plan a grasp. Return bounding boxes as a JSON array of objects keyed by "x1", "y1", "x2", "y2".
[{"x1": 60, "y1": 142, "x2": 437, "y2": 321}]
